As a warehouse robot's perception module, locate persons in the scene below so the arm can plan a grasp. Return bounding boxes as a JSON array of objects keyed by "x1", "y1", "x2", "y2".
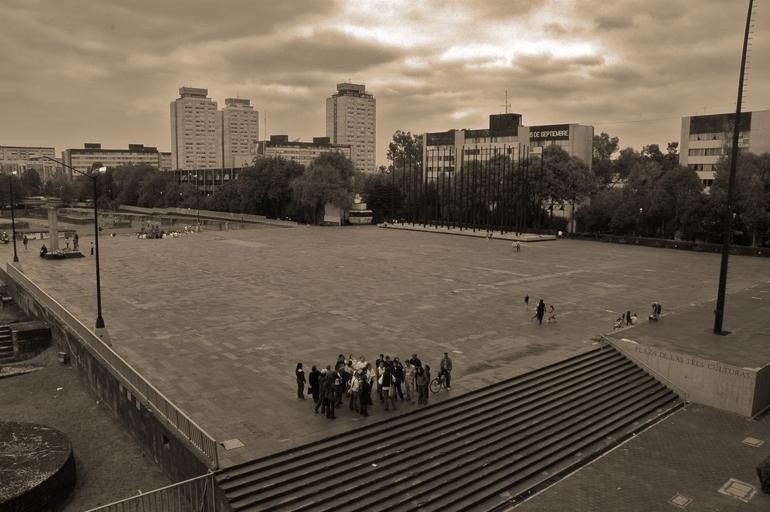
[
  {"x1": 517, "y1": 240, "x2": 522, "y2": 252},
  {"x1": 618, "y1": 311, "x2": 622, "y2": 319},
  {"x1": 548, "y1": 304, "x2": 558, "y2": 321},
  {"x1": 613, "y1": 318, "x2": 624, "y2": 329},
  {"x1": 23, "y1": 234, "x2": 29, "y2": 251},
  {"x1": 486, "y1": 231, "x2": 494, "y2": 241},
  {"x1": 622, "y1": 311, "x2": 626, "y2": 327},
  {"x1": 629, "y1": 312, "x2": 639, "y2": 325},
  {"x1": 536, "y1": 298, "x2": 548, "y2": 325},
  {"x1": 72, "y1": 234, "x2": 81, "y2": 251},
  {"x1": 626, "y1": 310, "x2": 633, "y2": 328},
  {"x1": 652, "y1": 302, "x2": 662, "y2": 320},
  {"x1": 295, "y1": 347, "x2": 454, "y2": 421},
  {"x1": 134, "y1": 220, "x2": 196, "y2": 241},
  {"x1": 531, "y1": 302, "x2": 548, "y2": 320},
  {"x1": 39, "y1": 243, "x2": 49, "y2": 258},
  {"x1": 89, "y1": 240, "x2": 95, "y2": 256},
  {"x1": 524, "y1": 291, "x2": 530, "y2": 311},
  {"x1": 511, "y1": 239, "x2": 517, "y2": 252},
  {"x1": 64, "y1": 236, "x2": 72, "y2": 249}
]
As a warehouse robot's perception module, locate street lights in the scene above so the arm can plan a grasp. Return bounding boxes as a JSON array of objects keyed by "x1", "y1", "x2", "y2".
[
  {"x1": 26, "y1": 154, "x2": 108, "y2": 329},
  {"x1": 7, "y1": 170, "x2": 18, "y2": 262},
  {"x1": 193, "y1": 175, "x2": 199, "y2": 224}
]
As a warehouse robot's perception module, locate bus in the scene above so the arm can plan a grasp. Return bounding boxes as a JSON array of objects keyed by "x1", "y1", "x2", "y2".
[{"x1": 348, "y1": 210, "x2": 374, "y2": 224}]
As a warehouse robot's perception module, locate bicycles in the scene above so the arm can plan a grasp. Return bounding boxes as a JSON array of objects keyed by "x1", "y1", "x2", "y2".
[{"x1": 429, "y1": 370, "x2": 451, "y2": 394}]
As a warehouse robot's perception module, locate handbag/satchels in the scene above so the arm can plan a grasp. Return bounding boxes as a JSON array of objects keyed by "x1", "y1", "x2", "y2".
[{"x1": 307, "y1": 387, "x2": 312, "y2": 394}]
